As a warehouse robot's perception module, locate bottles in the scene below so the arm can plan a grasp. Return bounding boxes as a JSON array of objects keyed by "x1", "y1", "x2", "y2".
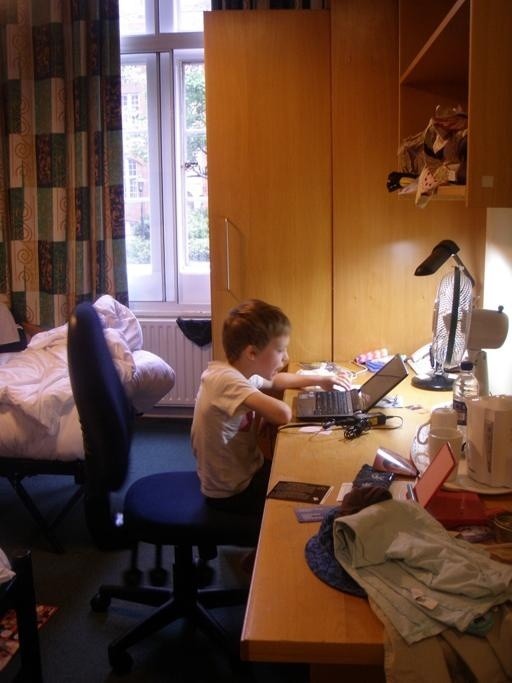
[{"x1": 452, "y1": 362, "x2": 479, "y2": 442}]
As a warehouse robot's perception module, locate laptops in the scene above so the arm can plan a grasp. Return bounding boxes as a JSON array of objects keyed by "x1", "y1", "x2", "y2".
[{"x1": 296, "y1": 351, "x2": 409, "y2": 420}]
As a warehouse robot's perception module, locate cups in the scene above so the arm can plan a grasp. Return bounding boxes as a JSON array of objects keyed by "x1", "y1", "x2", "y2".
[
  {"x1": 416, "y1": 408, "x2": 458, "y2": 445},
  {"x1": 428, "y1": 428, "x2": 464, "y2": 483}
]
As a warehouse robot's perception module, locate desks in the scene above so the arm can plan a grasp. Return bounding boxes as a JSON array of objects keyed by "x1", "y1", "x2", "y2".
[{"x1": 238, "y1": 359, "x2": 512, "y2": 667}]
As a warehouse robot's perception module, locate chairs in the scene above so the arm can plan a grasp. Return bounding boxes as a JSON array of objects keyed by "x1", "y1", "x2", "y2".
[{"x1": 68, "y1": 302, "x2": 265, "y2": 667}]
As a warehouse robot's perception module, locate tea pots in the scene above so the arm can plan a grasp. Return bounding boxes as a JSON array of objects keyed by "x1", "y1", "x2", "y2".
[{"x1": 462, "y1": 394, "x2": 512, "y2": 488}]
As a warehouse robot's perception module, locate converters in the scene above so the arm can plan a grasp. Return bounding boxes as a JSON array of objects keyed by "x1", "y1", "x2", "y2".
[{"x1": 355, "y1": 412, "x2": 386, "y2": 426}]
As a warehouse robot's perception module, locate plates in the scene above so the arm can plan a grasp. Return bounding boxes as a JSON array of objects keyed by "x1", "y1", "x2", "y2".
[{"x1": 411, "y1": 426, "x2": 512, "y2": 495}]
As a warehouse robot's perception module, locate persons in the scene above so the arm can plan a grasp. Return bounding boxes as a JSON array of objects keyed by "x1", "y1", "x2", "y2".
[{"x1": 190, "y1": 299, "x2": 352, "y2": 607}]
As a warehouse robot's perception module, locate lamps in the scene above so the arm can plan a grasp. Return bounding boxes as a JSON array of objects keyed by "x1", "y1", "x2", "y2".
[{"x1": 412, "y1": 239, "x2": 476, "y2": 391}]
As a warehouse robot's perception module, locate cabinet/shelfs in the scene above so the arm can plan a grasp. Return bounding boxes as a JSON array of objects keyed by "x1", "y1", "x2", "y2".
[
  {"x1": 1, "y1": 547, "x2": 61, "y2": 678},
  {"x1": 203, "y1": 7, "x2": 485, "y2": 363},
  {"x1": 399, "y1": 0, "x2": 511, "y2": 210}
]
